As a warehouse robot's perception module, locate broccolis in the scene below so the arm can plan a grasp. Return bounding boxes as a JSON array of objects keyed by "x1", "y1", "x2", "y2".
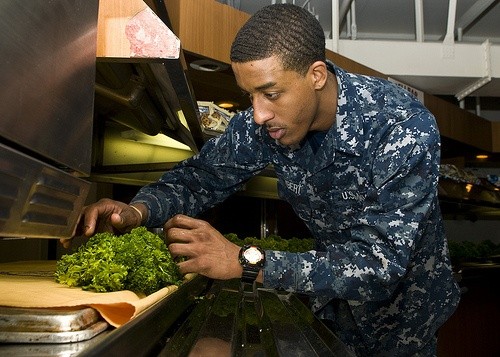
[
  {"x1": 55, "y1": 227, "x2": 183, "y2": 292},
  {"x1": 447, "y1": 238, "x2": 499, "y2": 259},
  {"x1": 225, "y1": 230, "x2": 319, "y2": 252}
]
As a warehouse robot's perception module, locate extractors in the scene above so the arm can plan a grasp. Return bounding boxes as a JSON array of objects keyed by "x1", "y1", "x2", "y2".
[{"x1": 96, "y1": 0, "x2": 206, "y2": 157}]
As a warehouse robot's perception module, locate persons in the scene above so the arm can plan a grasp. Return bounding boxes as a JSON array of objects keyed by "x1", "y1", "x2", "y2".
[{"x1": 58, "y1": 0, "x2": 461, "y2": 357}]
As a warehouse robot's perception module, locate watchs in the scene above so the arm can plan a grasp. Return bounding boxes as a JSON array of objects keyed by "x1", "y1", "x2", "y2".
[{"x1": 238, "y1": 244, "x2": 266, "y2": 285}]
